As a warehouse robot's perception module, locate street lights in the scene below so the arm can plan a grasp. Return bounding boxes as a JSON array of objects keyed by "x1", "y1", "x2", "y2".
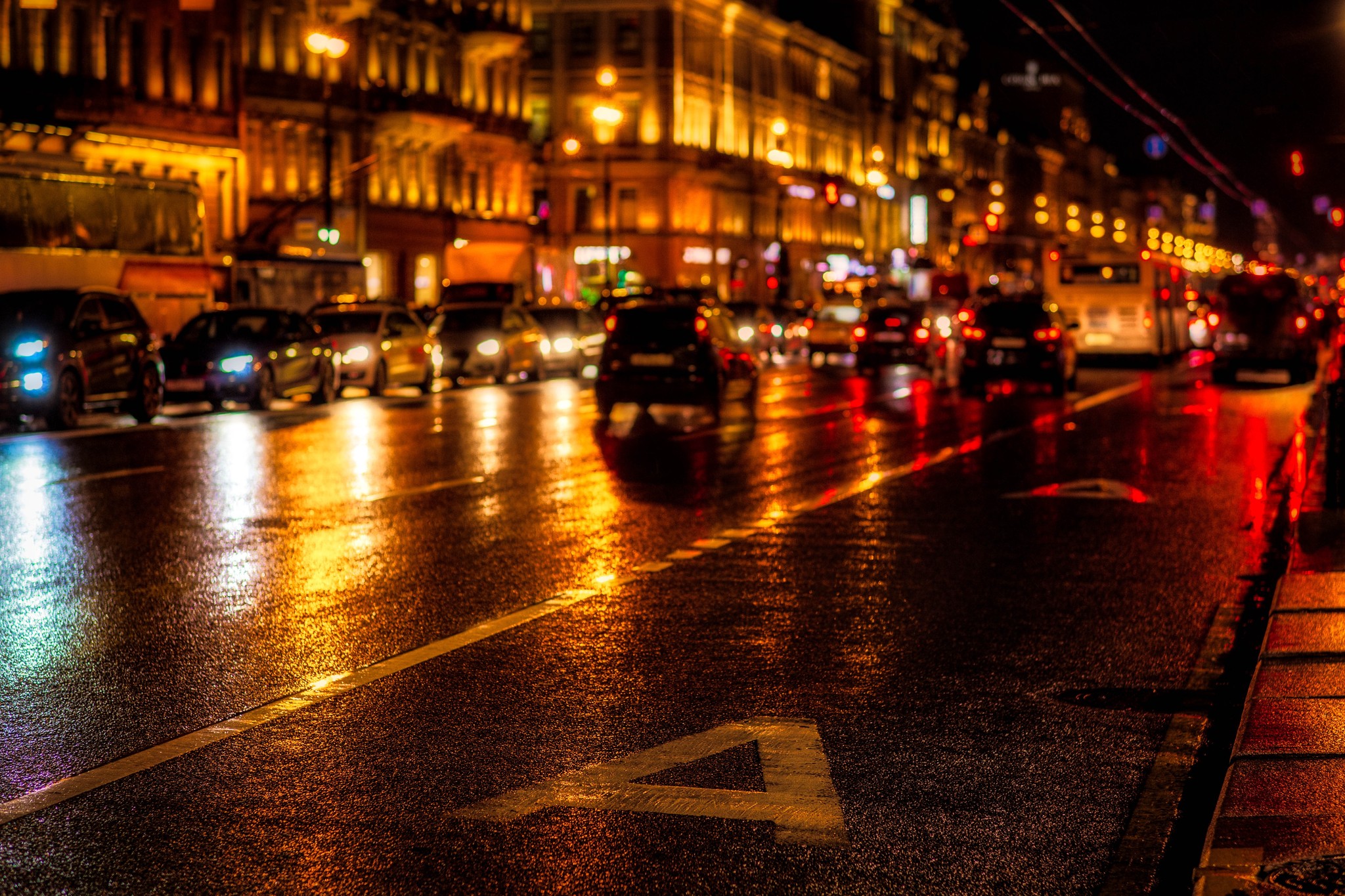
[
  {"x1": 304, "y1": 29, "x2": 349, "y2": 230},
  {"x1": 595, "y1": 63, "x2": 624, "y2": 285}
]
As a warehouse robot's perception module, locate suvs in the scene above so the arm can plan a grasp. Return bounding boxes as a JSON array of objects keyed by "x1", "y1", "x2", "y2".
[
  {"x1": 960, "y1": 301, "x2": 1079, "y2": 397},
  {"x1": 0, "y1": 286, "x2": 164, "y2": 431},
  {"x1": 1210, "y1": 274, "x2": 1317, "y2": 383},
  {"x1": 593, "y1": 285, "x2": 663, "y2": 316},
  {"x1": 662, "y1": 285, "x2": 720, "y2": 305},
  {"x1": 595, "y1": 302, "x2": 758, "y2": 414},
  {"x1": 436, "y1": 281, "x2": 525, "y2": 304},
  {"x1": 856, "y1": 305, "x2": 945, "y2": 373}
]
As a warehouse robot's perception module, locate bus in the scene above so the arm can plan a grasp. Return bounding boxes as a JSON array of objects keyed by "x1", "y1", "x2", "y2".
[{"x1": 1042, "y1": 241, "x2": 1188, "y2": 355}]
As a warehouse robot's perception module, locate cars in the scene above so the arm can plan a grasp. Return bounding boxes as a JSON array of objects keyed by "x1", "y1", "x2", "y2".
[
  {"x1": 753, "y1": 309, "x2": 782, "y2": 354},
  {"x1": 305, "y1": 305, "x2": 434, "y2": 396},
  {"x1": 426, "y1": 301, "x2": 549, "y2": 386},
  {"x1": 157, "y1": 307, "x2": 335, "y2": 411},
  {"x1": 724, "y1": 302, "x2": 755, "y2": 356},
  {"x1": 528, "y1": 303, "x2": 606, "y2": 379},
  {"x1": 809, "y1": 303, "x2": 869, "y2": 360},
  {"x1": 0, "y1": 359, "x2": 21, "y2": 433}
]
